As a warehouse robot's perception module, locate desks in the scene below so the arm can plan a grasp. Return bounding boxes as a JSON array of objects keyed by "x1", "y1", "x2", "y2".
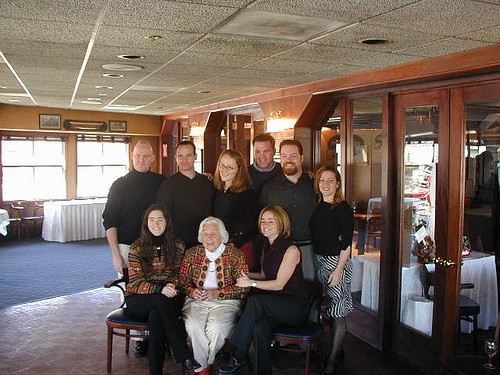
[
  {"x1": 41, "y1": 198, "x2": 108, "y2": 242},
  {"x1": 0, "y1": 208, "x2": 11, "y2": 236},
  {"x1": 355, "y1": 249, "x2": 498, "y2": 334},
  {"x1": 353, "y1": 213, "x2": 382, "y2": 254}
]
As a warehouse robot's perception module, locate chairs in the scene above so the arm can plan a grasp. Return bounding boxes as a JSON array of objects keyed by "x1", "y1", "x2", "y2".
[
  {"x1": 104, "y1": 267, "x2": 171, "y2": 374},
  {"x1": 4, "y1": 201, "x2": 44, "y2": 240},
  {"x1": 269, "y1": 282, "x2": 327, "y2": 375},
  {"x1": 419, "y1": 265, "x2": 481, "y2": 358}
]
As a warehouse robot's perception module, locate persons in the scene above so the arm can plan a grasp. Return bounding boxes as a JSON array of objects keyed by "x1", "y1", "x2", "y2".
[
  {"x1": 178, "y1": 217, "x2": 251, "y2": 375},
  {"x1": 246, "y1": 134, "x2": 283, "y2": 195},
  {"x1": 257, "y1": 139, "x2": 318, "y2": 371},
  {"x1": 102, "y1": 139, "x2": 214, "y2": 359},
  {"x1": 124, "y1": 203, "x2": 202, "y2": 375},
  {"x1": 156, "y1": 140, "x2": 220, "y2": 249},
  {"x1": 308, "y1": 166, "x2": 355, "y2": 375},
  {"x1": 213, "y1": 149, "x2": 256, "y2": 361},
  {"x1": 468, "y1": 151, "x2": 500, "y2": 256},
  {"x1": 217, "y1": 203, "x2": 309, "y2": 375}
]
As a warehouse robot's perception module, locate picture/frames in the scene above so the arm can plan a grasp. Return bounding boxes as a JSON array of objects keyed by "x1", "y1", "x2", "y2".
[
  {"x1": 108, "y1": 120, "x2": 126, "y2": 133},
  {"x1": 39, "y1": 114, "x2": 61, "y2": 130}
]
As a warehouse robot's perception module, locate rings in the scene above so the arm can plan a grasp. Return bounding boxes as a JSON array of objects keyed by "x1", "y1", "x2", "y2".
[
  {"x1": 204, "y1": 296, "x2": 206, "y2": 298},
  {"x1": 207, "y1": 298, "x2": 209, "y2": 300},
  {"x1": 170, "y1": 293, "x2": 172, "y2": 295}
]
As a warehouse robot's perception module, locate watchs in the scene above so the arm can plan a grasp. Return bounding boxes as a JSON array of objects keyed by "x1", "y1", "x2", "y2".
[{"x1": 252, "y1": 280, "x2": 257, "y2": 287}]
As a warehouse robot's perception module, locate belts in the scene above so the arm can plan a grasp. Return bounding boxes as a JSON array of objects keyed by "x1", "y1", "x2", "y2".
[{"x1": 291, "y1": 240, "x2": 312, "y2": 244}]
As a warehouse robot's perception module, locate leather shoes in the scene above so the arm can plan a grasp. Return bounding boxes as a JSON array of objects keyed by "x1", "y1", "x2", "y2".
[{"x1": 183, "y1": 357, "x2": 201, "y2": 370}]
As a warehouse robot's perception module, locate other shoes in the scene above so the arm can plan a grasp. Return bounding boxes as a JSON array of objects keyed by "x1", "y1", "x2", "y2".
[
  {"x1": 218, "y1": 355, "x2": 246, "y2": 373},
  {"x1": 164, "y1": 343, "x2": 171, "y2": 356},
  {"x1": 216, "y1": 350, "x2": 225, "y2": 360},
  {"x1": 189, "y1": 365, "x2": 212, "y2": 375},
  {"x1": 319, "y1": 360, "x2": 337, "y2": 375},
  {"x1": 326, "y1": 348, "x2": 345, "y2": 362},
  {"x1": 301, "y1": 350, "x2": 314, "y2": 365},
  {"x1": 134, "y1": 341, "x2": 146, "y2": 356}
]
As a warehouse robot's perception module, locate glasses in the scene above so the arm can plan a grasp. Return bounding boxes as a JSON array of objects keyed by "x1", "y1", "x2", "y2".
[{"x1": 220, "y1": 165, "x2": 238, "y2": 170}]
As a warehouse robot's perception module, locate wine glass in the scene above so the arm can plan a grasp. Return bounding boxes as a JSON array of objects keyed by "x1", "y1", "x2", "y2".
[{"x1": 482, "y1": 338, "x2": 498, "y2": 369}]
